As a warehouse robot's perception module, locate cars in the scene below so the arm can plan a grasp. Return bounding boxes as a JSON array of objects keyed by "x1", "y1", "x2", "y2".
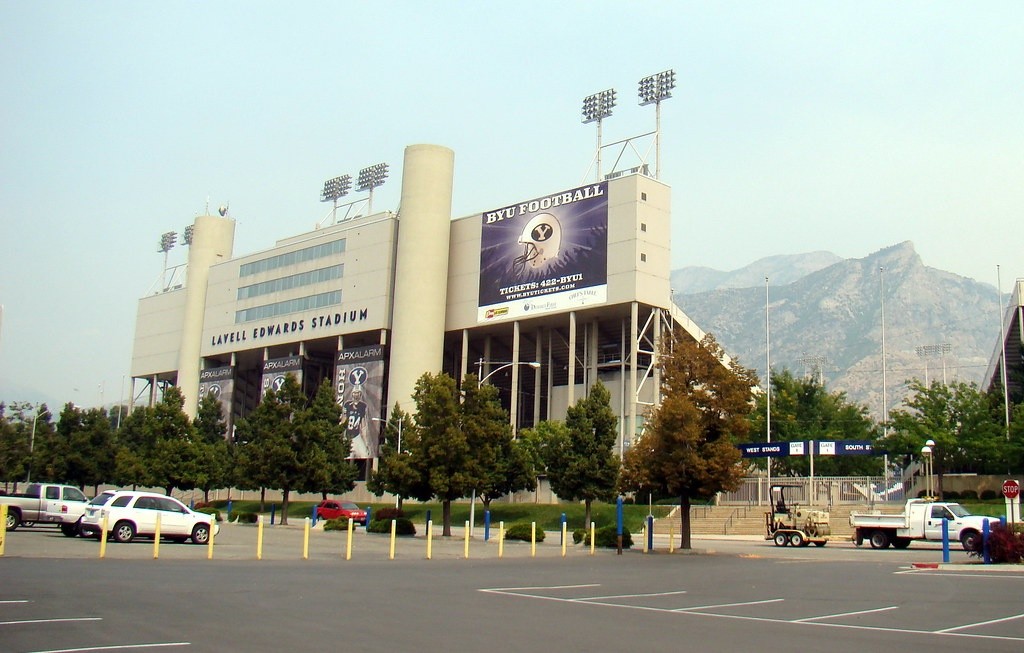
[{"x1": 316, "y1": 499, "x2": 367, "y2": 526}]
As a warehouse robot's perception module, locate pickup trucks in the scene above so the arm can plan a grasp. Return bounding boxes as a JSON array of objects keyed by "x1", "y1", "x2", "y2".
[
  {"x1": 0, "y1": 482, "x2": 92, "y2": 537},
  {"x1": 849, "y1": 496, "x2": 1001, "y2": 551}
]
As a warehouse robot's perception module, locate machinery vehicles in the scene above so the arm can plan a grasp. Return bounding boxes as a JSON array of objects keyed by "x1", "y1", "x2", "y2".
[{"x1": 764, "y1": 483, "x2": 831, "y2": 548}]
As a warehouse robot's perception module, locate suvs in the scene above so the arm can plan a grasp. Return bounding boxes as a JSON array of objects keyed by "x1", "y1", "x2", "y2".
[{"x1": 81, "y1": 489, "x2": 220, "y2": 545}]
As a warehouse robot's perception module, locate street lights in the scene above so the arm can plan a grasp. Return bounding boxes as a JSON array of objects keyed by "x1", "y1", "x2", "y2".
[
  {"x1": 157, "y1": 230, "x2": 177, "y2": 292},
  {"x1": 924, "y1": 439, "x2": 936, "y2": 497},
  {"x1": 582, "y1": 89, "x2": 618, "y2": 183},
  {"x1": 920, "y1": 446, "x2": 932, "y2": 496},
  {"x1": 320, "y1": 174, "x2": 353, "y2": 225},
  {"x1": 470, "y1": 357, "x2": 542, "y2": 537},
  {"x1": 355, "y1": 163, "x2": 390, "y2": 216},
  {"x1": 371, "y1": 416, "x2": 402, "y2": 511},
  {"x1": 637, "y1": 69, "x2": 676, "y2": 180},
  {"x1": 26, "y1": 406, "x2": 64, "y2": 482}
]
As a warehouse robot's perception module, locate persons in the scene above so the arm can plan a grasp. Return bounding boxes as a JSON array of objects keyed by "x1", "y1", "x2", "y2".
[{"x1": 341, "y1": 385, "x2": 370, "y2": 454}]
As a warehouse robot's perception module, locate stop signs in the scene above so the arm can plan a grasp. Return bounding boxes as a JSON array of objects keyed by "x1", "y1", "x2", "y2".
[{"x1": 1002, "y1": 479, "x2": 1020, "y2": 500}]
